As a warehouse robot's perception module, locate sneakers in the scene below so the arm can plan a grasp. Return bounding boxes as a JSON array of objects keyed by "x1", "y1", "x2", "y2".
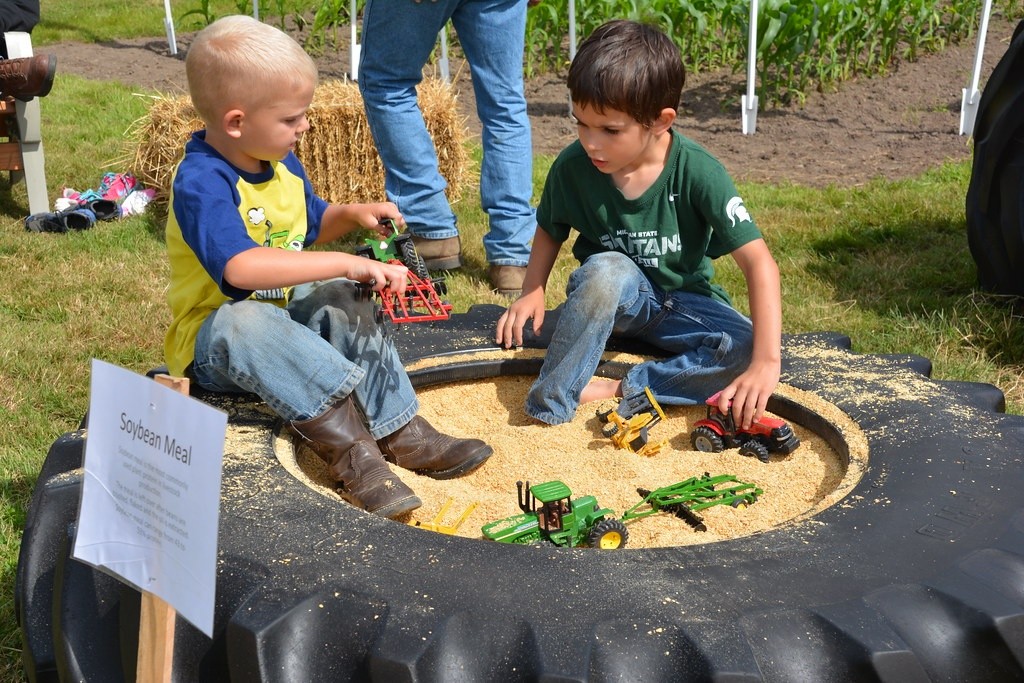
[
  {"x1": 489, "y1": 265, "x2": 527, "y2": 293},
  {"x1": 408, "y1": 228, "x2": 461, "y2": 270}
]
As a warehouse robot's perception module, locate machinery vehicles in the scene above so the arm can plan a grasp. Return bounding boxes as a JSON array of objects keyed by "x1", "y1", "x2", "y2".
[
  {"x1": 597, "y1": 387, "x2": 672, "y2": 461},
  {"x1": 357, "y1": 219, "x2": 430, "y2": 281},
  {"x1": 688, "y1": 391, "x2": 800, "y2": 464}
]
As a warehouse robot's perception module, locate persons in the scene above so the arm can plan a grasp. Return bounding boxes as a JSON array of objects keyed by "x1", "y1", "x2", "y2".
[
  {"x1": 163, "y1": 15, "x2": 492, "y2": 519},
  {"x1": 495, "y1": 20, "x2": 782, "y2": 431},
  {"x1": 357, "y1": 0, "x2": 537, "y2": 296}
]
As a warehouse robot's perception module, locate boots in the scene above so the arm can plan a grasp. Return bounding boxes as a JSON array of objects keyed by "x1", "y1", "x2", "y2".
[
  {"x1": 377, "y1": 413, "x2": 494, "y2": 481},
  {"x1": 290, "y1": 396, "x2": 423, "y2": 522}
]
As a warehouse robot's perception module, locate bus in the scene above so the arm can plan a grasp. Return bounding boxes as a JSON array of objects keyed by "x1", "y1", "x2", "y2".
[{"x1": 481, "y1": 478, "x2": 629, "y2": 550}]
